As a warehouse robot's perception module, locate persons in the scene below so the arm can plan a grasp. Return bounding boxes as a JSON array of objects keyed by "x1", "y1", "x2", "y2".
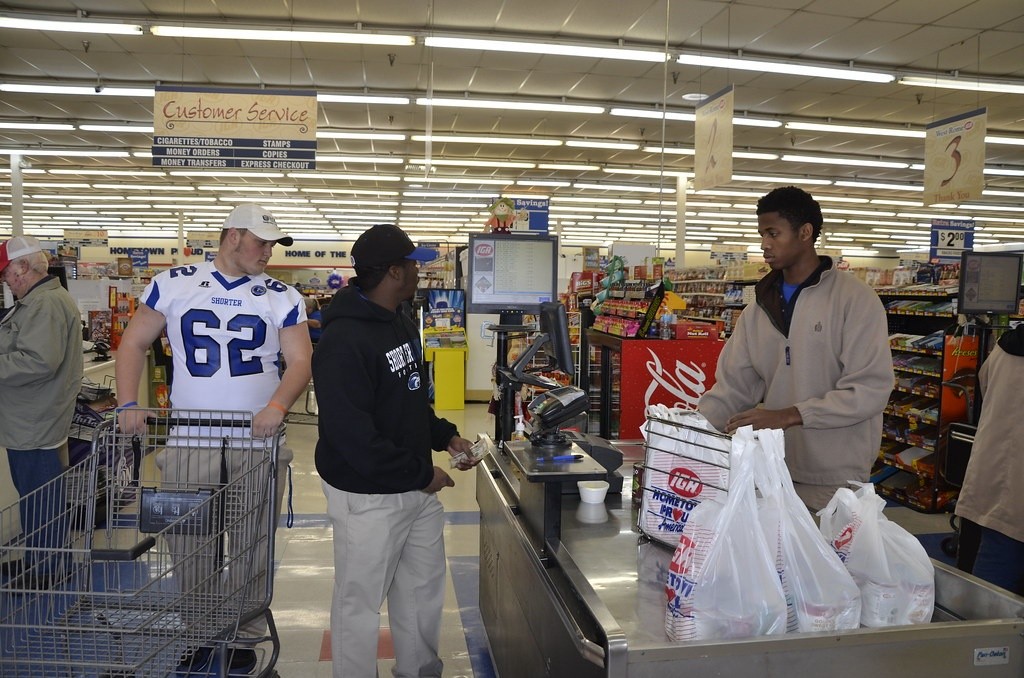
[
  {"x1": 304, "y1": 297, "x2": 322, "y2": 342},
  {"x1": 694, "y1": 185, "x2": 896, "y2": 529},
  {"x1": 115, "y1": 202, "x2": 314, "y2": 672},
  {"x1": 152, "y1": 325, "x2": 174, "y2": 416},
  {"x1": 954, "y1": 324, "x2": 1024, "y2": 593},
  {"x1": 311, "y1": 224, "x2": 481, "y2": 678},
  {"x1": 0, "y1": 236, "x2": 83, "y2": 598}
]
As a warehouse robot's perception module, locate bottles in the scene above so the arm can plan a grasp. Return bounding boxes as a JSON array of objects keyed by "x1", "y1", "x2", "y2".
[
  {"x1": 659, "y1": 306, "x2": 671, "y2": 340},
  {"x1": 113, "y1": 292, "x2": 130, "y2": 336}
]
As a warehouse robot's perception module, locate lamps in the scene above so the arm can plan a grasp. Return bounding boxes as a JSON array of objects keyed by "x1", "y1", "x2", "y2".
[{"x1": 0, "y1": 8, "x2": 1024, "y2": 257}]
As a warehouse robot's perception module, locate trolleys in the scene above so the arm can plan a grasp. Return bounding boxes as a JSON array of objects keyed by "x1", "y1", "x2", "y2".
[{"x1": 1, "y1": 401, "x2": 287, "y2": 678}]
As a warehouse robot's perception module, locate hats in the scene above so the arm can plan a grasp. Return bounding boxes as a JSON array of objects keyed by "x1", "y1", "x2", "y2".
[
  {"x1": 350, "y1": 223, "x2": 438, "y2": 275},
  {"x1": 223, "y1": 203, "x2": 293, "y2": 247},
  {"x1": 0, "y1": 235, "x2": 43, "y2": 273}
]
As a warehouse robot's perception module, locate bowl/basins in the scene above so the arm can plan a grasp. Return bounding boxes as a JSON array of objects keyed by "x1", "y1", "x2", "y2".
[
  {"x1": 577, "y1": 480, "x2": 610, "y2": 504},
  {"x1": 577, "y1": 501, "x2": 608, "y2": 524}
]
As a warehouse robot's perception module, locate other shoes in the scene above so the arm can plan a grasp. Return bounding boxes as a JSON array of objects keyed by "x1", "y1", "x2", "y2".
[
  {"x1": 175, "y1": 645, "x2": 215, "y2": 674},
  {"x1": 226, "y1": 646, "x2": 257, "y2": 676},
  {"x1": 8, "y1": 569, "x2": 73, "y2": 591}
]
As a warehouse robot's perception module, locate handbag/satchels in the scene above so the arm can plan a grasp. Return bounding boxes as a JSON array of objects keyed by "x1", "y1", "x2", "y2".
[{"x1": 639, "y1": 404, "x2": 935, "y2": 644}]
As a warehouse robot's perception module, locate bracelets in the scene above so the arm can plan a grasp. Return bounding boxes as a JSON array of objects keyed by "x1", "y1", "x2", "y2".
[
  {"x1": 117, "y1": 402, "x2": 138, "y2": 414},
  {"x1": 267, "y1": 402, "x2": 288, "y2": 415}
]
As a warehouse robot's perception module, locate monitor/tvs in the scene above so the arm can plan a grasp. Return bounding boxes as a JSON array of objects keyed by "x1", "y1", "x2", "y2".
[
  {"x1": 539, "y1": 301, "x2": 575, "y2": 376},
  {"x1": 958, "y1": 251, "x2": 1021, "y2": 314},
  {"x1": 467, "y1": 232, "x2": 558, "y2": 314}
]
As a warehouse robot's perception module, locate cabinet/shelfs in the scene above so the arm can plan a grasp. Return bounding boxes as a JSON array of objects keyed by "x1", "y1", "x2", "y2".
[
  {"x1": 878, "y1": 292, "x2": 959, "y2": 325},
  {"x1": 667, "y1": 265, "x2": 755, "y2": 337},
  {"x1": 872, "y1": 328, "x2": 984, "y2": 515},
  {"x1": 110, "y1": 292, "x2": 135, "y2": 350},
  {"x1": 580, "y1": 274, "x2": 665, "y2": 336},
  {"x1": 523, "y1": 313, "x2": 583, "y2": 404}
]
